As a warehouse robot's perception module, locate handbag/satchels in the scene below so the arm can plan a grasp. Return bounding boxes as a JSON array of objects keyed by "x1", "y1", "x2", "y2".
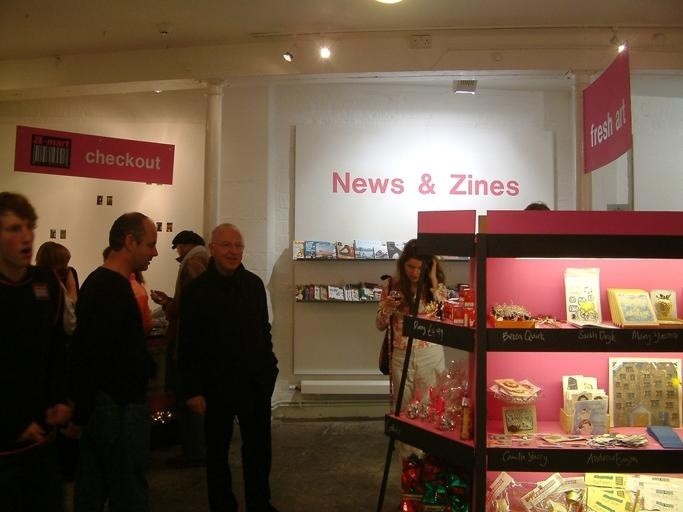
[{"x1": 378, "y1": 330, "x2": 392, "y2": 376}]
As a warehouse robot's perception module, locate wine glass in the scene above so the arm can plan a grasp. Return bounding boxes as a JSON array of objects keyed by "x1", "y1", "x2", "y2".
[{"x1": 389, "y1": 290, "x2": 403, "y2": 314}]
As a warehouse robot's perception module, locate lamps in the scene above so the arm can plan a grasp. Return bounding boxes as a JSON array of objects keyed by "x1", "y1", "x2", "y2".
[
  {"x1": 283, "y1": 35, "x2": 297, "y2": 63},
  {"x1": 452, "y1": 79, "x2": 478, "y2": 94},
  {"x1": 319, "y1": 31, "x2": 331, "y2": 59}
]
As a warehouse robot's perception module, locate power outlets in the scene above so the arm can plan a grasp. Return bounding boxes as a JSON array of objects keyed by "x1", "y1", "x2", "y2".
[{"x1": 410, "y1": 35, "x2": 433, "y2": 49}]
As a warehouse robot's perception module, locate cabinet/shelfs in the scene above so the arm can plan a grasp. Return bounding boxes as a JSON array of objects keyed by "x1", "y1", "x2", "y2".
[
  {"x1": 292, "y1": 258, "x2": 398, "y2": 304},
  {"x1": 376, "y1": 234, "x2": 683, "y2": 512}
]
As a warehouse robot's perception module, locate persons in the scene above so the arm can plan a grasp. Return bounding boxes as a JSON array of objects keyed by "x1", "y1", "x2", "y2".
[
  {"x1": 524, "y1": 200, "x2": 552, "y2": 212},
  {"x1": 34, "y1": 240, "x2": 80, "y2": 483},
  {"x1": 0, "y1": 191, "x2": 83, "y2": 511},
  {"x1": 375, "y1": 238, "x2": 447, "y2": 469},
  {"x1": 67, "y1": 211, "x2": 159, "y2": 510},
  {"x1": 148, "y1": 227, "x2": 209, "y2": 467},
  {"x1": 175, "y1": 222, "x2": 283, "y2": 512},
  {"x1": 100, "y1": 244, "x2": 153, "y2": 332}
]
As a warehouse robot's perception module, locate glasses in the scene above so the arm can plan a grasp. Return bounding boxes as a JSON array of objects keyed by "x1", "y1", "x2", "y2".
[{"x1": 210, "y1": 242, "x2": 246, "y2": 250}]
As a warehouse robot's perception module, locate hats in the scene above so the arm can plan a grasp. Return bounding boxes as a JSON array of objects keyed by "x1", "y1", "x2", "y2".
[{"x1": 171, "y1": 230, "x2": 205, "y2": 249}]
